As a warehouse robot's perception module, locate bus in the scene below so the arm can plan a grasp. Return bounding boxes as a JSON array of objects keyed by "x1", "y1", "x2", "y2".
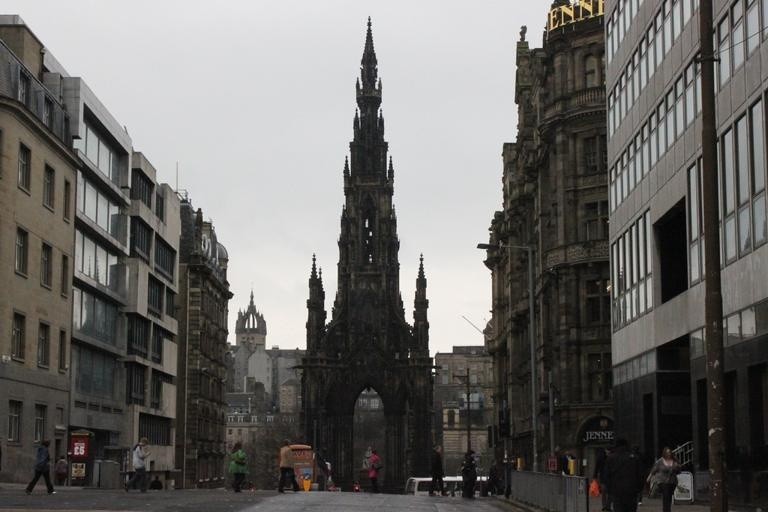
[
  {"x1": 405, "y1": 475, "x2": 491, "y2": 497},
  {"x1": 279, "y1": 445, "x2": 332, "y2": 490}
]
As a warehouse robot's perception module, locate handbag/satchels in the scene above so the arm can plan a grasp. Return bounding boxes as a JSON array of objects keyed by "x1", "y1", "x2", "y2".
[{"x1": 235, "y1": 458, "x2": 246, "y2": 464}]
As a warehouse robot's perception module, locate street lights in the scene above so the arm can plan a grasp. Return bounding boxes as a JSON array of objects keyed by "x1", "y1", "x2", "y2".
[{"x1": 477, "y1": 243, "x2": 538, "y2": 462}]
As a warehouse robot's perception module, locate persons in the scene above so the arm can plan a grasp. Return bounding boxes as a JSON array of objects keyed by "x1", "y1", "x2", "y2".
[
  {"x1": 55, "y1": 455, "x2": 68, "y2": 486},
  {"x1": 646, "y1": 447, "x2": 682, "y2": 512},
  {"x1": 429, "y1": 445, "x2": 450, "y2": 496},
  {"x1": 592, "y1": 447, "x2": 611, "y2": 512},
  {"x1": 229, "y1": 441, "x2": 249, "y2": 492},
  {"x1": 278, "y1": 439, "x2": 300, "y2": 494},
  {"x1": 366, "y1": 450, "x2": 383, "y2": 493},
  {"x1": 24, "y1": 439, "x2": 57, "y2": 495},
  {"x1": 246, "y1": 480, "x2": 256, "y2": 490},
  {"x1": 149, "y1": 475, "x2": 163, "y2": 489},
  {"x1": 352, "y1": 481, "x2": 360, "y2": 492},
  {"x1": 603, "y1": 437, "x2": 643, "y2": 512},
  {"x1": 123, "y1": 437, "x2": 152, "y2": 492},
  {"x1": 490, "y1": 460, "x2": 497, "y2": 480},
  {"x1": 461, "y1": 451, "x2": 477, "y2": 500}
]
